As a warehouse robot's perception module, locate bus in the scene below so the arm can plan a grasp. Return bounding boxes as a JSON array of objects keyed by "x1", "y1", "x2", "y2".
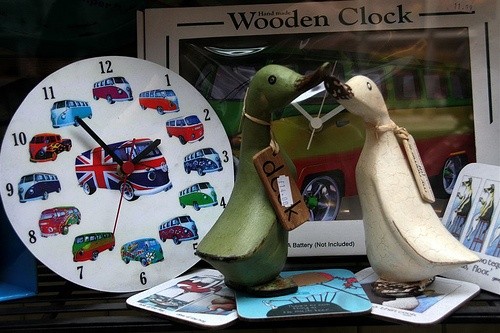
[
  {"x1": 71, "y1": 232, "x2": 115, "y2": 262},
  {"x1": 138, "y1": 89, "x2": 180, "y2": 115},
  {"x1": 50, "y1": 99, "x2": 92, "y2": 128},
  {"x1": 178, "y1": 182, "x2": 218, "y2": 211},
  {"x1": 17, "y1": 172, "x2": 61, "y2": 203},
  {"x1": 166, "y1": 114, "x2": 204, "y2": 145},
  {"x1": 120, "y1": 238, "x2": 164, "y2": 267},
  {"x1": 93, "y1": 77, "x2": 133, "y2": 104},
  {"x1": 38, "y1": 206, "x2": 82, "y2": 235},
  {"x1": 159, "y1": 215, "x2": 199, "y2": 245},
  {"x1": 184, "y1": 147, "x2": 223, "y2": 176},
  {"x1": 75, "y1": 138, "x2": 172, "y2": 202}
]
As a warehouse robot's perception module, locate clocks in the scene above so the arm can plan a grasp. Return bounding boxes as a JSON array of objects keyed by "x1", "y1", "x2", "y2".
[
  {"x1": 135, "y1": 0, "x2": 500, "y2": 256},
  {"x1": 0, "y1": 56, "x2": 235, "y2": 293}
]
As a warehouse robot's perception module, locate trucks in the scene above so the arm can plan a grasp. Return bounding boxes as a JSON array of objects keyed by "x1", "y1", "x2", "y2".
[{"x1": 28, "y1": 133, "x2": 72, "y2": 163}]
